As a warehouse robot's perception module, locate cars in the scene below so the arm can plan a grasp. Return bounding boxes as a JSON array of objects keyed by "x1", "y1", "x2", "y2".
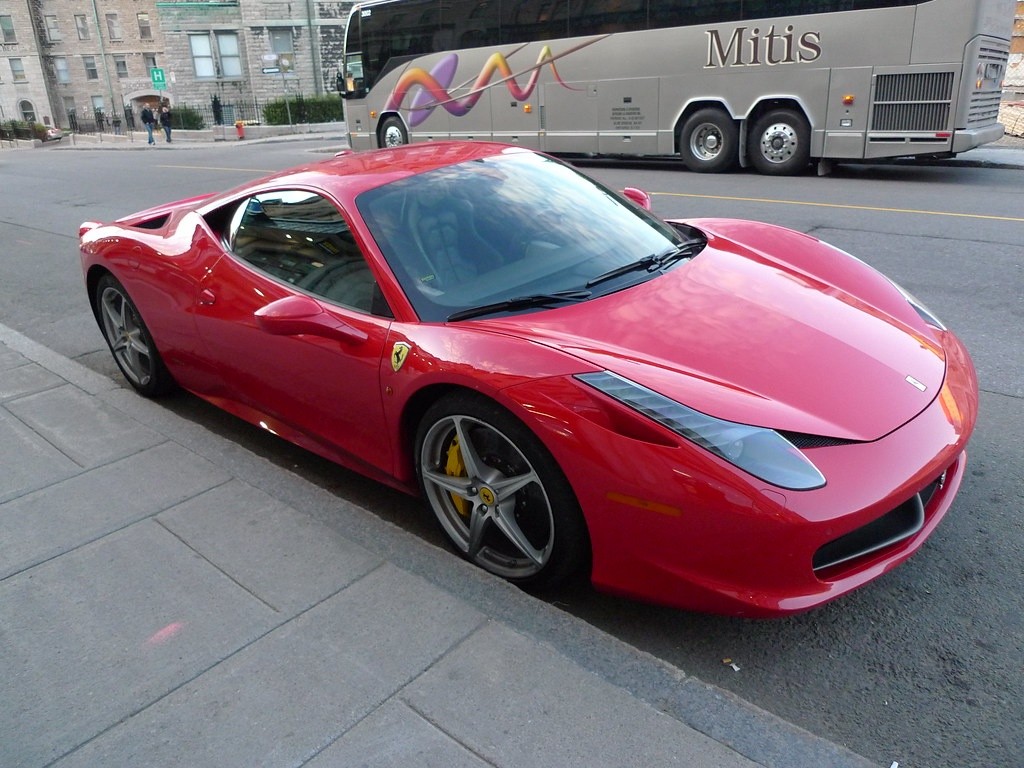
[{"x1": 43, "y1": 124, "x2": 62, "y2": 142}]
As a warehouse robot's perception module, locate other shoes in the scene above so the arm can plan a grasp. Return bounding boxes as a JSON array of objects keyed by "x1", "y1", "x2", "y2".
[
  {"x1": 167, "y1": 139, "x2": 171, "y2": 143},
  {"x1": 149, "y1": 142, "x2": 155, "y2": 145}
]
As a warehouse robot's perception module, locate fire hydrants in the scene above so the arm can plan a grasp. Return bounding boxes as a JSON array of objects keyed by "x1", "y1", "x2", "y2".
[{"x1": 233, "y1": 122, "x2": 245, "y2": 139}]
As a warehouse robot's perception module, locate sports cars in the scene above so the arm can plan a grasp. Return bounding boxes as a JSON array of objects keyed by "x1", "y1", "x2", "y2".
[{"x1": 80, "y1": 136, "x2": 977, "y2": 621}]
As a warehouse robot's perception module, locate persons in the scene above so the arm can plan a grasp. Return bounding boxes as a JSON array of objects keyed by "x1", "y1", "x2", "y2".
[
  {"x1": 140, "y1": 103, "x2": 156, "y2": 146},
  {"x1": 159, "y1": 107, "x2": 174, "y2": 143}
]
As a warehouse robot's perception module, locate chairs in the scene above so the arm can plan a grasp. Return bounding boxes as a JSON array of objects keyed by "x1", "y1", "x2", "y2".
[
  {"x1": 295, "y1": 255, "x2": 374, "y2": 313},
  {"x1": 396, "y1": 174, "x2": 506, "y2": 291}
]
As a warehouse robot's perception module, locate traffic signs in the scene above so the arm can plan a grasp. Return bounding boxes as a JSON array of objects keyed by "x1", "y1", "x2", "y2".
[{"x1": 261, "y1": 67, "x2": 279, "y2": 73}]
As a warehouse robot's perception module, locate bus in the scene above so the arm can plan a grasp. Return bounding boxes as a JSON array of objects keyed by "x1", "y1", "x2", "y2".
[{"x1": 335, "y1": 0, "x2": 1024, "y2": 179}]
